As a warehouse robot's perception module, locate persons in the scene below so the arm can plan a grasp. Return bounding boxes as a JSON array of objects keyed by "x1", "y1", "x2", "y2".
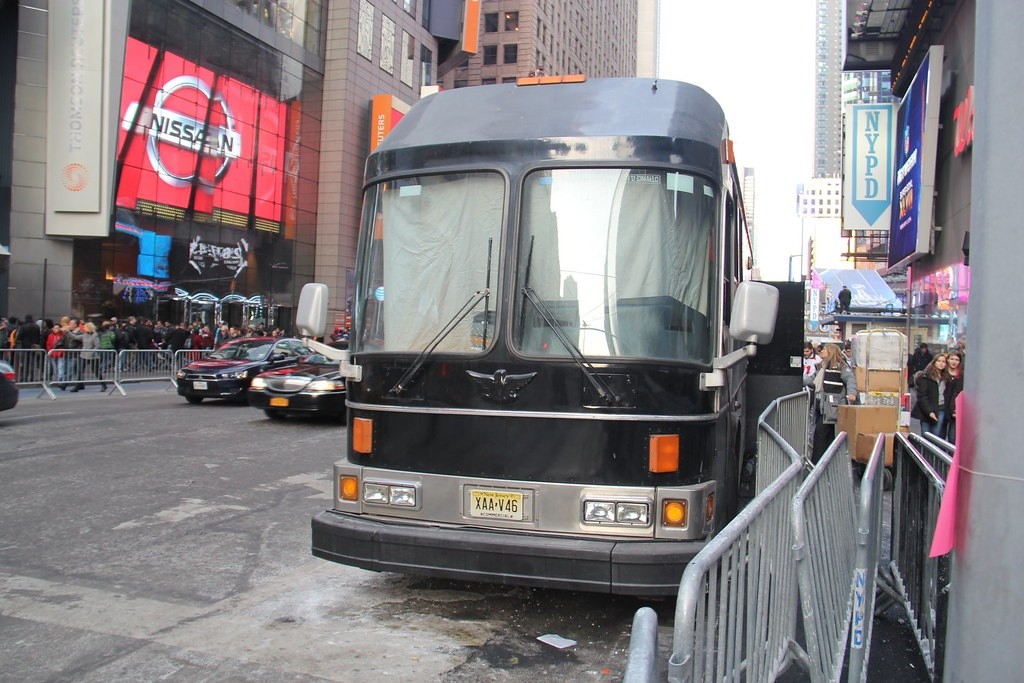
[
  {"x1": 911, "y1": 350, "x2": 965, "y2": 457},
  {"x1": 838, "y1": 286, "x2": 851, "y2": 314},
  {"x1": 0, "y1": 315, "x2": 286, "y2": 392},
  {"x1": 803, "y1": 341, "x2": 856, "y2": 464},
  {"x1": 909, "y1": 343, "x2": 933, "y2": 393}
]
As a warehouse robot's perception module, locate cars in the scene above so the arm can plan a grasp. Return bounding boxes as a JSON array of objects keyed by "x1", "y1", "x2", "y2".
[
  {"x1": 177, "y1": 335, "x2": 316, "y2": 406},
  {"x1": 247, "y1": 337, "x2": 349, "y2": 427}
]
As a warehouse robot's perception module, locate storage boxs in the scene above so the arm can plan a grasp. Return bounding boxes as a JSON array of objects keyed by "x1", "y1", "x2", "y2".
[{"x1": 838, "y1": 331, "x2": 912, "y2": 467}]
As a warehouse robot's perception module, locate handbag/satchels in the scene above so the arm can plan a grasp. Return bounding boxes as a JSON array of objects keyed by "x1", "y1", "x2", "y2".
[{"x1": 823, "y1": 391, "x2": 847, "y2": 425}]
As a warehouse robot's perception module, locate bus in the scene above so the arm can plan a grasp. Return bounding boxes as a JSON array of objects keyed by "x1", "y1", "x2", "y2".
[{"x1": 310, "y1": 73, "x2": 781, "y2": 600}]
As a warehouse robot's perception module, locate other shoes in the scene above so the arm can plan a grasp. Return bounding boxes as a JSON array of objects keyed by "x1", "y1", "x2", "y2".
[
  {"x1": 70, "y1": 385, "x2": 85, "y2": 392},
  {"x1": 58, "y1": 385, "x2": 65, "y2": 391},
  {"x1": 101, "y1": 386, "x2": 107, "y2": 392}
]
